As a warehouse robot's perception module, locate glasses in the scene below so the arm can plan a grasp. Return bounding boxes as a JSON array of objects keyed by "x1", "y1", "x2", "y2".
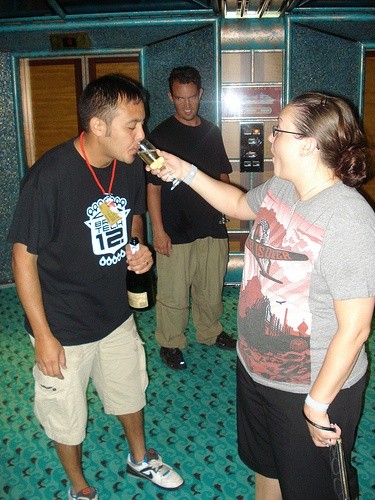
[{"x1": 272, "y1": 125, "x2": 309, "y2": 138}]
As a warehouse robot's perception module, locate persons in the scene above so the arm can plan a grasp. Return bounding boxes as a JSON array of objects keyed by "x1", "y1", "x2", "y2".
[
  {"x1": 144, "y1": 65, "x2": 237, "y2": 369},
  {"x1": 145, "y1": 92, "x2": 375, "y2": 500},
  {"x1": 6, "y1": 74, "x2": 183, "y2": 500}
]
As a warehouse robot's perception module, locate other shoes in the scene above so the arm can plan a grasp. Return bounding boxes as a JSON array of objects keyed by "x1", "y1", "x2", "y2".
[
  {"x1": 160, "y1": 346, "x2": 187, "y2": 370},
  {"x1": 215, "y1": 331, "x2": 237, "y2": 350}
]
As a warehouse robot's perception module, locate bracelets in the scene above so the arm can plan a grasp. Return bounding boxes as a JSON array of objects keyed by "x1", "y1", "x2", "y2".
[
  {"x1": 304, "y1": 394, "x2": 330, "y2": 411},
  {"x1": 184, "y1": 163, "x2": 198, "y2": 185}
]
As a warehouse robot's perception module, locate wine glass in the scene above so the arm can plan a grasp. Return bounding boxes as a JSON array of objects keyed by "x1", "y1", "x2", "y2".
[{"x1": 134, "y1": 139, "x2": 183, "y2": 190}]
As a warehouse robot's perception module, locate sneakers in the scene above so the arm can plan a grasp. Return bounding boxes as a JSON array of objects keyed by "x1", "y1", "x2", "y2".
[
  {"x1": 67, "y1": 486, "x2": 98, "y2": 500},
  {"x1": 126, "y1": 448, "x2": 185, "y2": 491}
]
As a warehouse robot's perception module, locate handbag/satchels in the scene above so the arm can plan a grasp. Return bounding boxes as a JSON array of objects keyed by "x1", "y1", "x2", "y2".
[{"x1": 302, "y1": 410, "x2": 349, "y2": 500}]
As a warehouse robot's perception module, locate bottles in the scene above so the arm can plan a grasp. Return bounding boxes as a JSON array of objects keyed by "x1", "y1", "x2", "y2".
[{"x1": 126, "y1": 236, "x2": 156, "y2": 311}]
